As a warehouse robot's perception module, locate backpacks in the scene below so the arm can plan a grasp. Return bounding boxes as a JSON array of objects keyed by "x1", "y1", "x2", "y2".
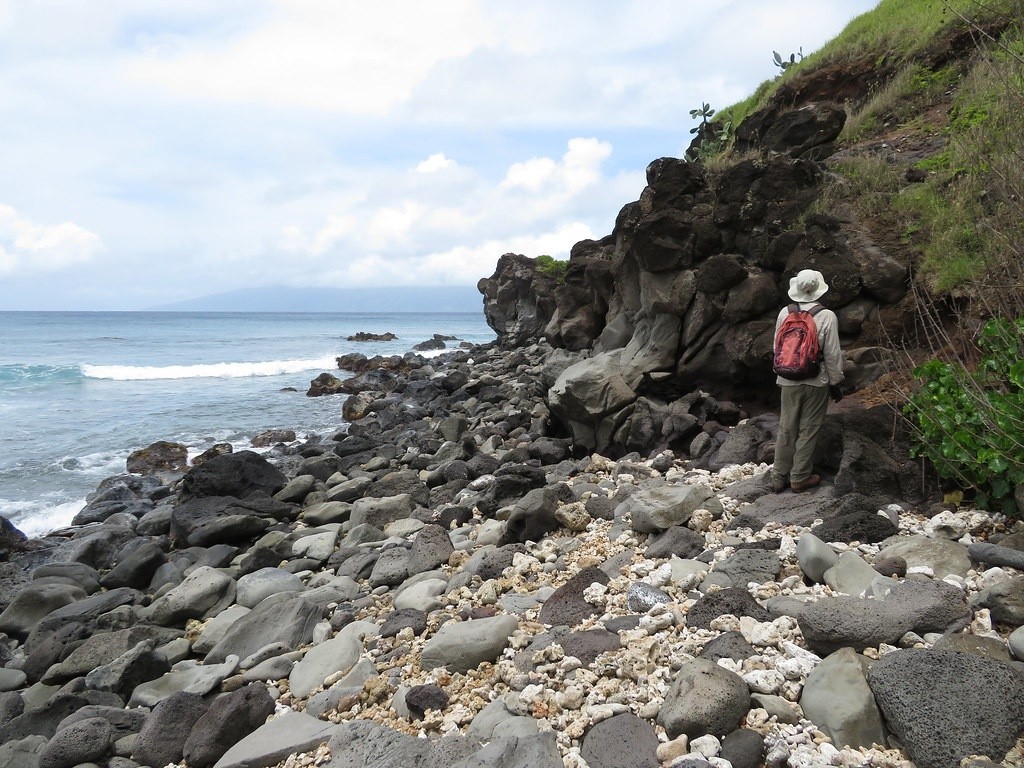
[{"x1": 773, "y1": 303, "x2": 828, "y2": 380}]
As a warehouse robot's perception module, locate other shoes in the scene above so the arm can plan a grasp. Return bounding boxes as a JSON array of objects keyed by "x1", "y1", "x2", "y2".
[
  {"x1": 791, "y1": 475, "x2": 820, "y2": 493},
  {"x1": 772, "y1": 481, "x2": 791, "y2": 494}
]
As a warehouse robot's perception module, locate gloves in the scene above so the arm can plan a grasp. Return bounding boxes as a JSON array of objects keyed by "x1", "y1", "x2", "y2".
[{"x1": 829, "y1": 385, "x2": 843, "y2": 403}]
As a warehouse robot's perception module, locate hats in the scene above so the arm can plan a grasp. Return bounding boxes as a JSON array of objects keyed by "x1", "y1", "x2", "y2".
[{"x1": 788, "y1": 269, "x2": 829, "y2": 302}]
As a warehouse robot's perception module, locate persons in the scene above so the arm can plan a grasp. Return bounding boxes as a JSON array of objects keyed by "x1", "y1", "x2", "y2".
[{"x1": 771, "y1": 269, "x2": 843, "y2": 492}]
